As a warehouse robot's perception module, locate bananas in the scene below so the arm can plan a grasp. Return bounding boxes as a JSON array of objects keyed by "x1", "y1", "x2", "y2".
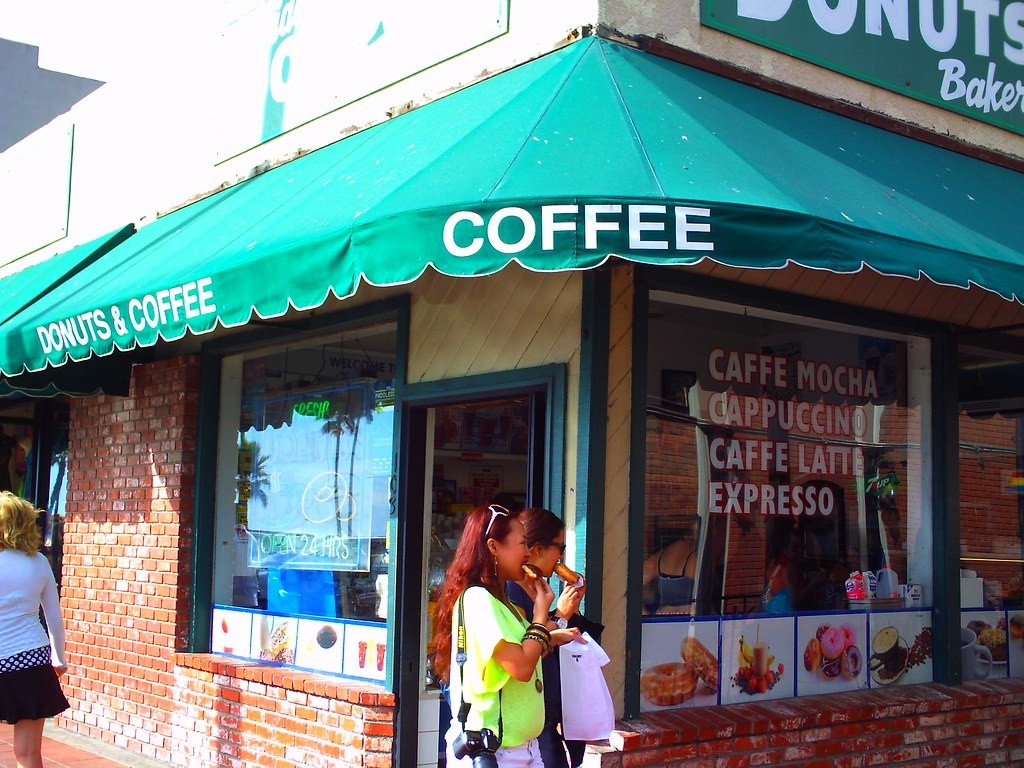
[{"x1": 737, "y1": 634, "x2": 775, "y2": 669}]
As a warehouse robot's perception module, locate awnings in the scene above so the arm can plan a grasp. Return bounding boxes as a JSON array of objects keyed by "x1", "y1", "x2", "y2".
[{"x1": 0, "y1": 33, "x2": 1024, "y2": 404}]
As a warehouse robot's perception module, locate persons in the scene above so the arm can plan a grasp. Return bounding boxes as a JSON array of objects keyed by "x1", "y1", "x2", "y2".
[
  {"x1": 503, "y1": 508, "x2": 605, "y2": 768},
  {"x1": 434, "y1": 504, "x2": 590, "y2": 767},
  {"x1": 639, "y1": 507, "x2": 710, "y2": 617},
  {"x1": 760, "y1": 532, "x2": 807, "y2": 616},
  {"x1": 0, "y1": 490, "x2": 73, "y2": 767}
]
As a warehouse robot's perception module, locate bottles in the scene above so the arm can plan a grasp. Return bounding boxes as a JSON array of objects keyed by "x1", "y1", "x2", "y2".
[{"x1": 875, "y1": 565, "x2": 898, "y2": 599}]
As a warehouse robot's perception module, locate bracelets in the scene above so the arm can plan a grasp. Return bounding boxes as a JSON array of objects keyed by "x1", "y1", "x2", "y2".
[{"x1": 521, "y1": 623, "x2": 554, "y2": 657}]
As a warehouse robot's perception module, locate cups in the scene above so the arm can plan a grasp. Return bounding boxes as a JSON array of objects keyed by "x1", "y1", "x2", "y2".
[
  {"x1": 869, "y1": 627, "x2": 900, "y2": 671},
  {"x1": 961, "y1": 627, "x2": 992, "y2": 680}
]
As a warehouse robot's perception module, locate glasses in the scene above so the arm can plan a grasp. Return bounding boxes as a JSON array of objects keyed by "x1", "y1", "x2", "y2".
[
  {"x1": 484, "y1": 504, "x2": 510, "y2": 549},
  {"x1": 542, "y1": 541, "x2": 566, "y2": 553}
]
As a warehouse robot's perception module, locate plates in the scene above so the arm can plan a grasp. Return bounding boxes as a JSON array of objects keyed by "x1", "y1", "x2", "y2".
[
  {"x1": 871, "y1": 635, "x2": 908, "y2": 685},
  {"x1": 977, "y1": 657, "x2": 1008, "y2": 665}
]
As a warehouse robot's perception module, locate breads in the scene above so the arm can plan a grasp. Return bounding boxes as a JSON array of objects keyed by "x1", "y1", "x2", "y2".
[{"x1": 553, "y1": 563, "x2": 578, "y2": 583}]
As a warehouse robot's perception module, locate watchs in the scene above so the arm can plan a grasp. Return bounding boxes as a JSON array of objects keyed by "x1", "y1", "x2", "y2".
[{"x1": 549, "y1": 615, "x2": 569, "y2": 630}]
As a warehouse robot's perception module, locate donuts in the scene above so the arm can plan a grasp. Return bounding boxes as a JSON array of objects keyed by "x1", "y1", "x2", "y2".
[
  {"x1": 803, "y1": 622, "x2": 862, "y2": 681},
  {"x1": 639, "y1": 636, "x2": 718, "y2": 706}
]
